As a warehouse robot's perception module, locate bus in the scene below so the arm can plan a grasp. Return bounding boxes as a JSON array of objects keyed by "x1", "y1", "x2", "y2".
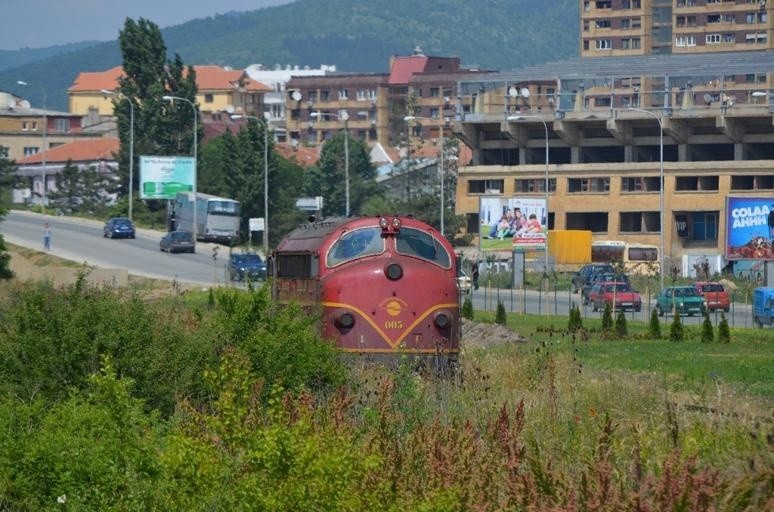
[
  {"x1": 269, "y1": 212, "x2": 464, "y2": 383},
  {"x1": 172, "y1": 191, "x2": 242, "y2": 242}
]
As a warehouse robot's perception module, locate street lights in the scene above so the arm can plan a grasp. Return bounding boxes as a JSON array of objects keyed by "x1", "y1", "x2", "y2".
[
  {"x1": 99, "y1": 90, "x2": 133, "y2": 220},
  {"x1": 626, "y1": 105, "x2": 665, "y2": 289},
  {"x1": 16, "y1": 81, "x2": 46, "y2": 213},
  {"x1": 507, "y1": 113, "x2": 550, "y2": 271},
  {"x1": 162, "y1": 95, "x2": 197, "y2": 239},
  {"x1": 402, "y1": 114, "x2": 444, "y2": 236},
  {"x1": 311, "y1": 111, "x2": 350, "y2": 216},
  {"x1": 231, "y1": 115, "x2": 269, "y2": 253}
]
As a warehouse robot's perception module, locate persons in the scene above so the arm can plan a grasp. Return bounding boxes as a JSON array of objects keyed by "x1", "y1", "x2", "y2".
[
  {"x1": 480, "y1": 208, "x2": 516, "y2": 241},
  {"x1": 512, "y1": 210, "x2": 526, "y2": 237},
  {"x1": 523, "y1": 213, "x2": 544, "y2": 233},
  {"x1": 41, "y1": 223, "x2": 52, "y2": 251},
  {"x1": 494, "y1": 215, "x2": 510, "y2": 241}
]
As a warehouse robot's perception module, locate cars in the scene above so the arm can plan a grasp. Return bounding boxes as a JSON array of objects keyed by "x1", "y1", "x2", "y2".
[
  {"x1": 229, "y1": 252, "x2": 267, "y2": 282},
  {"x1": 567, "y1": 260, "x2": 642, "y2": 315},
  {"x1": 655, "y1": 280, "x2": 732, "y2": 317},
  {"x1": 102, "y1": 218, "x2": 134, "y2": 239},
  {"x1": 456, "y1": 268, "x2": 472, "y2": 294},
  {"x1": 159, "y1": 231, "x2": 194, "y2": 253}
]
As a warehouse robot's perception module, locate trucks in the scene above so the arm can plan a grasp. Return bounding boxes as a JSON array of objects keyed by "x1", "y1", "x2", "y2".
[{"x1": 753, "y1": 287, "x2": 774, "y2": 327}]
